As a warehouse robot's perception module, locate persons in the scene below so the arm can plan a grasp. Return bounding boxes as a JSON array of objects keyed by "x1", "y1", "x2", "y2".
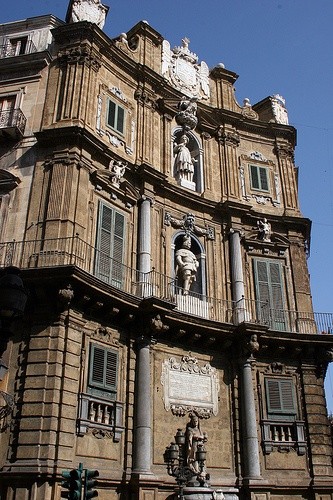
[
  {"x1": 111, "y1": 161, "x2": 128, "y2": 184},
  {"x1": 182, "y1": 95, "x2": 198, "y2": 114},
  {"x1": 186, "y1": 416, "x2": 207, "y2": 473},
  {"x1": 175, "y1": 235, "x2": 199, "y2": 295},
  {"x1": 173, "y1": 135, "x2": 198, "y2": 181},
  {"x1": 260, "y1": 217, "x2": 270, "y2": 240}
]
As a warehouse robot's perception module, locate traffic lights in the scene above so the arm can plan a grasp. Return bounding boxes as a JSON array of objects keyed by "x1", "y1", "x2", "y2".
[
  {"x1": 69, "y1": 469, "x2": 81, "y2": 500},
  {"x1": 60, "y1": 471, "x2": 70, "y2": 499},
  {"x1": 87, "y1": 470, "x2": 100, "y2": 500}
]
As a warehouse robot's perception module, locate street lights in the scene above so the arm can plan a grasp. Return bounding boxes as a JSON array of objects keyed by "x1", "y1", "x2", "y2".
[{"x1": 163, "y1": 427, "x2": 207, "y2": 500}]
